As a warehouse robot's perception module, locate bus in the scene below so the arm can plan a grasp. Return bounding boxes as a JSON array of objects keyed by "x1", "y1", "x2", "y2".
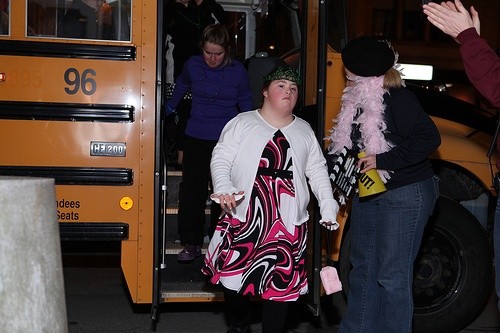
[{"x1": 0, "y1": 0, "x2": 500, "y2": 333}]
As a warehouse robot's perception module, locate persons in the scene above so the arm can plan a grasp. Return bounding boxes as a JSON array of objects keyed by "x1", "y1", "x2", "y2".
[
  {"x1": 162, "y1": 0, "x2": 228, "y2": 167},
  {"x1": 201, "y1": 64, "x2": 340, "y2": 333},
  {"x1": 422, "y1": 0, "x2": 500, "y2": 302},
  {"x1": 0, "y1": 0, "x2": 130, "y2": 42},
  {"x1": 322, "y1": 35, "x2": 441, "y2": 333},
  {"x1": 163, "y1": 24, "x2": 254, "y2": 260}
]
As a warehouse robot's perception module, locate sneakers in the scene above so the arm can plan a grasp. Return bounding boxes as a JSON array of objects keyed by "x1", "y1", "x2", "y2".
[{"x1": 176, "y1": 245, "x2": 201, "y2": 261}]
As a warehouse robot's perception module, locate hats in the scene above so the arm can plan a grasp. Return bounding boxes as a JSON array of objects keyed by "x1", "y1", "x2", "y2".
[{"x1": 343, "y1": 34, "x2": 396, "y2": 77}]
